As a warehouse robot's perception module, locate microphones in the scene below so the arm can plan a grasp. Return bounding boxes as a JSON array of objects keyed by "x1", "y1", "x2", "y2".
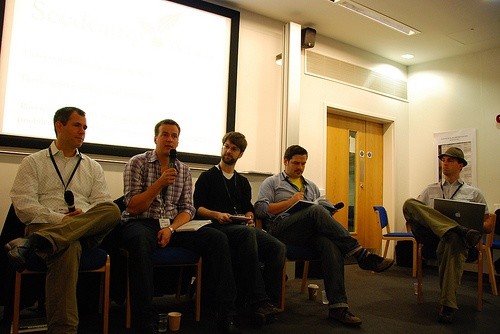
[
  {"x1": 170, "y1": 149, "x2": 177, "y2": 168},
  {"x1": 335, "y1": 202, "x2": 345, "y2": 209},
  {"x1": 64, "y1": 191, "x2": 75, "y2": 212}
]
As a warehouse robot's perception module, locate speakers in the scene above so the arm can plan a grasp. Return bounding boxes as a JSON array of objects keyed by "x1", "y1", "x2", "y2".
[{"x1": 302, "y1": 27, "x2": 316, "y2": 48}]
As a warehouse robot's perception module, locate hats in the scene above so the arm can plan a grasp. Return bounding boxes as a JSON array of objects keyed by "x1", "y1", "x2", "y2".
[{"x1": 438, "y1": 147, "x2": 468, "y2": 167}]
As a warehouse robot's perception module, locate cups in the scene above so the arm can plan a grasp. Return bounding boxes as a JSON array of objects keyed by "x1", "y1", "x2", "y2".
[
  {"x1": 322, "y1": 290, "x2": 329, "y2": 304},
  {"x1": 168, "y1": 312, "x2": 182, "y2": 331},
  {"x1": 158, "y1": 314, "x2": 168, "y2": 332},
  {"x1": 307, "y1": 283, "x2": 319, "y2": 302}
]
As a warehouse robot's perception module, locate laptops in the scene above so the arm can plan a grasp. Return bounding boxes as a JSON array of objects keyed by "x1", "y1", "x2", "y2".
[{"x1": 434, "y1": 199, "x2": 486, "y2": 234}]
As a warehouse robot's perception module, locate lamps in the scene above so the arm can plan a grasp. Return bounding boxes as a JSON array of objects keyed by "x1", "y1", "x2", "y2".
[{"x1": 275, "y1": 54, "x2": 282, "y2": 66}]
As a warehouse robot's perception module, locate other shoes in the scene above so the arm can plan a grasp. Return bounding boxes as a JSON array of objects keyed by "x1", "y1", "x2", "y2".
[
  {"x1": 5, "y1": 237, "x2": 32, "y2": 272},
  {"x1": 359, "y1": 253, "x2": 394, "y2": 272},
  {"x1": 219, "y1": 311, "x2": 239, "y2": 334},
  {"x1": 328, "y1": 307, "x2": 362, "y2": 325},
  {"x1": 251, "y1": 300, "x2": 282, "y2": 315},
  {"x1": 439, "y1": 304, "x2": 456, "y2": 321},
  {"x1": 465, "y1": 229, "x2": 481, "y2": 249}
]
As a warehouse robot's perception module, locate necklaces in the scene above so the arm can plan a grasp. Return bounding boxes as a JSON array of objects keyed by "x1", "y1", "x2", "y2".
[
  {"x1": 440, "y1": 182, "x2": 464, "y2": 199},
  {"x1": 282, "y1": 172, "x2": 308, "y2": 200}
]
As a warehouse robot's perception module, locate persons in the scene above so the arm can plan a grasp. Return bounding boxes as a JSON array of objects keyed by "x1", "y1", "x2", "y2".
[
  {"x1": 5, "y1": 107, "x2": 121, "y2": 334},
  {"x1": 253, "y1": 145, "x2": 394, "y2": 326},
  {"x1": 193, "y1": 132, "x2": 288, "y2": 334},
  {"x1": 403, "y1": 147, "x2": 494, "y2": 324},
  {"x1": 118, "y1": 119, "x2": 236, "y2": 334}
]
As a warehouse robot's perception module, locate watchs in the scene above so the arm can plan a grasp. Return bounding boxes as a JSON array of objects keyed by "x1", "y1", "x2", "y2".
[{"x1": 169, "y1": 225, "x2": 174, "y2": 235}]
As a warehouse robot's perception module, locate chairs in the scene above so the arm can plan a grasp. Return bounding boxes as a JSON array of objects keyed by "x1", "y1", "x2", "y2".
[
  {"x1": 11, "y1": 212, "x2": 311, "y2": 333},
  {"x1": 373, "y1": 206, "x2": 500, "y2": 311}
]
